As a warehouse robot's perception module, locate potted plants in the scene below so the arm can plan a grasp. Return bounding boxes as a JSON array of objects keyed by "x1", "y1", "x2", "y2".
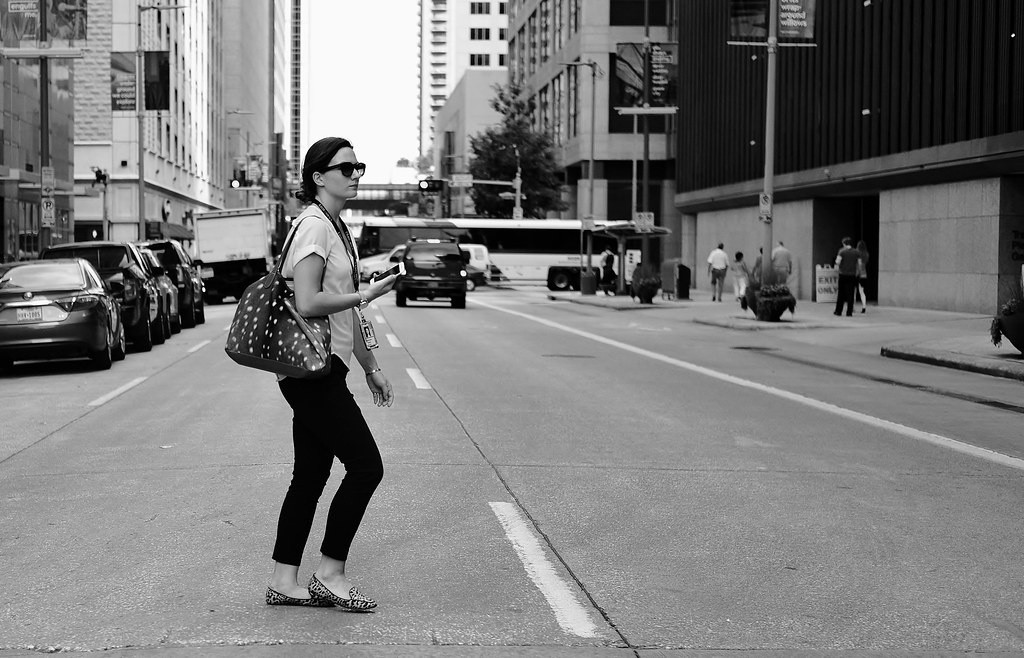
[
  {"x1": 990, "y1": 275, "x2": 1024, "y2": 360},
  {"x1": 630, "y1": 273, "x2": 663, "y2": 304},
  {"x1": 738, "y1": 261, "x2": 796, "y2": 322}
]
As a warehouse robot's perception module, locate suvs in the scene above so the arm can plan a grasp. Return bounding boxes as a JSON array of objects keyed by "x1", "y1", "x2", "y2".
[
  {"x1": 39, "y1": 241, "x2": 164, "y2": 352},
  {"x1": 142, "y1": 239, "x2": 205, "y2": 331},
  {"x1": 390, "y1": 237, "x2": 471, "y2": 308}
]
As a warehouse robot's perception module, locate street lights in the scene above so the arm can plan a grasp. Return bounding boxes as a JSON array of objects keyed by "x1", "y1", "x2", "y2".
[
  {"x1": 560, "y1": 62, "x2": 596, "y2": 265},
  {"x1": 140, "y1": 5, "x2": 186, "y2": 239},
  {"x1": 3, "y1": 46, "x2": 85, "y2": 250}
]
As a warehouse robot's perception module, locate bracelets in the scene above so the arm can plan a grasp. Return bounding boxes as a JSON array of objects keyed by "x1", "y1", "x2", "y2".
[{"x1": 366, "y1": 369, "x2": 381, "y2": 375}]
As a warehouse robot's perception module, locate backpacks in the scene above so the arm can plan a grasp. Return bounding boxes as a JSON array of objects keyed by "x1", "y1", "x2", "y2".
[{"x1": 604, "y1": 250, "x2": 614, "y2": 268}]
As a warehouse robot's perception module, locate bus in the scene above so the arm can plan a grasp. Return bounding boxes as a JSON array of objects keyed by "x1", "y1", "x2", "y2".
[{"x1": 358, "y1": 217, "x2": 648, "y2": 290}]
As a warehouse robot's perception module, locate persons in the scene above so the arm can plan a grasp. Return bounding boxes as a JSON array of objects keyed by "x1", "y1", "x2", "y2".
[
  {"x1": 853, "y1": 240, "x2": 869, "y2": 314},
  {"x1": 771, "y1": 241, "x2": 792, "y2": 286},
  {"x1": 832, "y1": 236, "x2": 862, "y2": 317},
  {"x1": 601, "y1": 246, "x2": 614, "y2": 296},
  {"x1": 730, "y1": 252, "x2": 751, "y2": 300},
  {"x1": 752, "y1": 247, "x2": 763, "y2": 285},
  {"x1": 707, "y1": 242, "x2": 730, "y2": 300},
  {"x1": 265, "y1": 137, "x2": 397, "y2": 610},
  {"x1": 364, "y1": 327, "x2": 371, "y2": 337}
]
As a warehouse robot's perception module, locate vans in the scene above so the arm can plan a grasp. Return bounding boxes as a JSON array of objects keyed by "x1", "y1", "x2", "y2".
[{"x1": 458, "y1": 243, "x2": 489, "y2": 279}]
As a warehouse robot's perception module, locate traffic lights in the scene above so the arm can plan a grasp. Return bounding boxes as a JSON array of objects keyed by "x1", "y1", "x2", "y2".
[{"x1": 230, "y1": 179, "x2": 253, "y2": 188}]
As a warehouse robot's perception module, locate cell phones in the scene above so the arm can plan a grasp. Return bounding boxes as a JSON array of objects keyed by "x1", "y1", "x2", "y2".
[{"x1": 370, "y1": 262, "x2": 405, "y2": 284}]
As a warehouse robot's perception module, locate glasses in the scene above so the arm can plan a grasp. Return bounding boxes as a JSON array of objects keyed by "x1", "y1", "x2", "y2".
[{"x1": 320, "y1": 162, "x2": 366, "y2": 177}]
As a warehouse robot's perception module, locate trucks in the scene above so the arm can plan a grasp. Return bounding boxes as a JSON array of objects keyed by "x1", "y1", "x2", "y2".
[{"x1": 192, "y1": 207, "x2": 273, "y2": 304}]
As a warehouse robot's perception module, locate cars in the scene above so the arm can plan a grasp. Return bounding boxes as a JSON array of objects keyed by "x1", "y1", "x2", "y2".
[
  {"x1": 466, "y1": 264, "x2": 485, "y2": 291},
  {"x1": 419, "y1": 180, "x2": 443, "y2": 190},
  {"x1": 137, "y1": 247, "x2": 181, "y2": 341},
  {"x1": 359, "y1": 245, "x2": 405, "y2": 279},
  {"x1": 0, "y1": 258, "x2": 125, "y2": 373}
]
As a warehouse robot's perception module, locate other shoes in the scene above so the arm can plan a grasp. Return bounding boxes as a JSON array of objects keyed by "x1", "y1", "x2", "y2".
[
  {"x1": 712, "y1": 296, "x2": 716, "y2": 302},
  {"x1": 265, "y1": 586, "x2": 334, "y2": 607},
  {"x1": 861, "y1": 307, "x2": 866, "y2": 313},
  {"x1": 308, "y1": 572, "x2": 377, "y2": 609}
]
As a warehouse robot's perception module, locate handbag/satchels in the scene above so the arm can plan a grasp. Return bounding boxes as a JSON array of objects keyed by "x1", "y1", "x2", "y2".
[{"x1": 224, "y1": 215, "x2": 331, "y2": 378}]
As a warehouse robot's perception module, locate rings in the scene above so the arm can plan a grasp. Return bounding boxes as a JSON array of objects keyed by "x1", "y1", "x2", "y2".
[{"x1": 373, "y1": 393, "x2": 377, "y2": 394}]
